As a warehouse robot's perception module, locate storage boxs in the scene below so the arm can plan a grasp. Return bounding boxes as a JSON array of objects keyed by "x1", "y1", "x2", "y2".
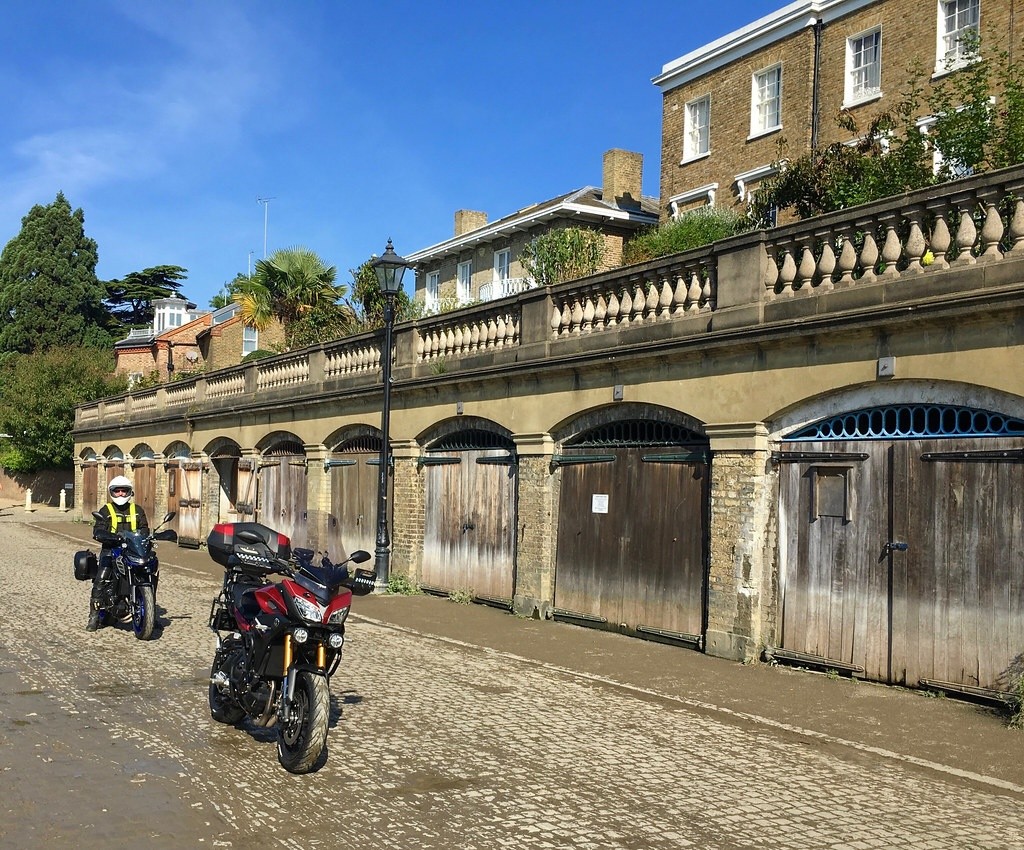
[
  {"x1": 206, "y1": 521, "x2": 291, "y2": 576},
  {"x1": 74, "y1": 551, "x2": 94, "y2": 581}
]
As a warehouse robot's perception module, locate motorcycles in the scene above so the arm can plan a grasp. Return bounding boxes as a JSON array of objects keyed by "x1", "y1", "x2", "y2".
[
  {"x1": 73, "y1": 510, "x2": 178, "y2": 641},
  {"x1": 204, "y1": 508, "x2": 377, "y2": 774}
]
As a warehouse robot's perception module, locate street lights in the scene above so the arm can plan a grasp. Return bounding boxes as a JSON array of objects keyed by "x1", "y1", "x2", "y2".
[{"x1": 366, "y1": 237, "x2": 410, "y2": 581}]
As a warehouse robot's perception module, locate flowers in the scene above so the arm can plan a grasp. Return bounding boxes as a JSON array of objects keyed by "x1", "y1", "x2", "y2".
[{"x1": 921, "y1": 249, "x2": 936, "y2": 265}]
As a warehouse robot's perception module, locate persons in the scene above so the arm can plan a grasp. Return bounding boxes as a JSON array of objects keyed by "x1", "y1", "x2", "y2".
[{"x1": 85, "y1": 476, "x2": 150, "y2": 632}]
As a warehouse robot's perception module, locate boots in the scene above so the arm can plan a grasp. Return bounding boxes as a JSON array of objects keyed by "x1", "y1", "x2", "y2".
[{"x1": 86, "y1": 598, "x2": 103, "y2": 631}]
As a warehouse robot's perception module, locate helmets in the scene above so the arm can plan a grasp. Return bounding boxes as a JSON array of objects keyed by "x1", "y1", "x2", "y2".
[{"x1": 108, "y1": 476, "x2": 133, "y2": 506}]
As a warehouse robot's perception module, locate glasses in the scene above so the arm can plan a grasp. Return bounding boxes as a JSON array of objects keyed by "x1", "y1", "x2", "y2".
[{"x1": 115, "y1": 489, "x2": 128, "y2": 493}]
